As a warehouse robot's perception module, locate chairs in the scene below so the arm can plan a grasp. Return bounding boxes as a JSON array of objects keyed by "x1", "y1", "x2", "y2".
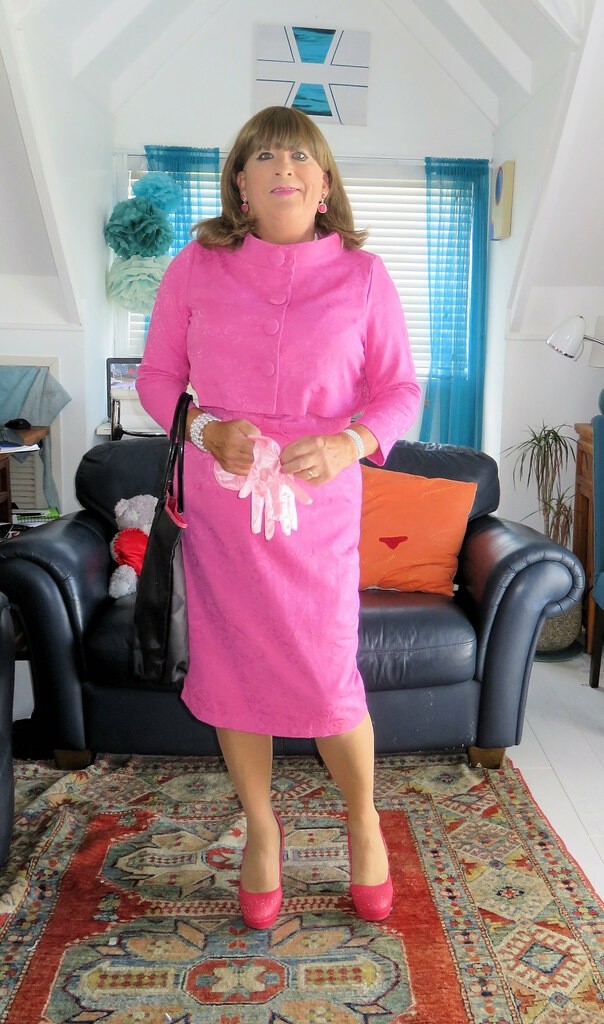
[
  {"x1": 108, "y1": 388, "x2": 200, "y2": 444},
  {"x1": 589, "y1": 388, "x2": 604, "y2": 688}
]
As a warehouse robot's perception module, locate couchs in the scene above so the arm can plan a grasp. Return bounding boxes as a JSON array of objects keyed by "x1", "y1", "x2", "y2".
[{"x1": 0, "y1": 437, "x2": 587, "y2": 770}]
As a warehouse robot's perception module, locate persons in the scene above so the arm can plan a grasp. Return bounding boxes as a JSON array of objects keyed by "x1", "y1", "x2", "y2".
[{"x1": 135, "y1": 106, "x2": 422, "y2": 930}]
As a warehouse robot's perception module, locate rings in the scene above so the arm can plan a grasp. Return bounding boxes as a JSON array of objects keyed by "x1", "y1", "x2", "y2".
[{"x1": 308, "y1": 470, "x2": 315, "y2": 478}]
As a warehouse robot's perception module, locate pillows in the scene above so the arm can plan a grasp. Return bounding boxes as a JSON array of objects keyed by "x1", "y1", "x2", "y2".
[{"x1": 358, "y1": 462, "x2": 478, "y2": 597}]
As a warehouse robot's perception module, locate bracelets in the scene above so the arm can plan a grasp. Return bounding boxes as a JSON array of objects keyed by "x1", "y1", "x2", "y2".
[
  {"x1": 190, "y1": 413, "x2": 221, "y2": 452},
  {"x1": 344, "y1": 428, "x2": 365, "y2": 458}
]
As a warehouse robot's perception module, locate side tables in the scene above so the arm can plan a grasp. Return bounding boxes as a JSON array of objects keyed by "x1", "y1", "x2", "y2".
[{"x1": 572, "y1": 422, "x2": 595, "y2": 655}]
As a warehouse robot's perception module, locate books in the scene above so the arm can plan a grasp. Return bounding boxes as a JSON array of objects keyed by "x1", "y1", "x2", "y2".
[{"x1": 12, "y1": 508, "x2": 59, "y2": 524}]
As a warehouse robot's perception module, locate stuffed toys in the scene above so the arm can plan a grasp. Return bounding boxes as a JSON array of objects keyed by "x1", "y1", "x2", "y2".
[{"x1": 110, "y1": 494, "x2": 159, "y2": 599}]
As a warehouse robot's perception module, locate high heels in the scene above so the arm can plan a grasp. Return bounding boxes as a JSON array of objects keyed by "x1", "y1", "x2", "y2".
[
  {"x1": 238, "y1": 810, "x2": 284, "y2": 929},
  {"x1": 347, "y1": 825, "x2": 394, "y2": 921}
]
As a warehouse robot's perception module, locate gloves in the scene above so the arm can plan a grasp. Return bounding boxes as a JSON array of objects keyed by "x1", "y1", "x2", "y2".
[{"x1": 213, "y1": 434, "x2": 313, "y2": 541}]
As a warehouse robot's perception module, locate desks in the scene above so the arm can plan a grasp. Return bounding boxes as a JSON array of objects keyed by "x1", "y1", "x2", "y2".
[{"x1": 0, "y1": 452, "x2": 14, "y2": 524}]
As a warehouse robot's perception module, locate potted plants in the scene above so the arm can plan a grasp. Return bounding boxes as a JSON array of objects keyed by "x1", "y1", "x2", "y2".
[{"x1": 494, "y1": 418, "x2": 583, "y2": 654}]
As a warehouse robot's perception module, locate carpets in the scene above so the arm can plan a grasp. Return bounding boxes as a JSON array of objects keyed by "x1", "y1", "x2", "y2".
[{"x1": 0, "y1": 752, "x2": 604, "y2": 1024}]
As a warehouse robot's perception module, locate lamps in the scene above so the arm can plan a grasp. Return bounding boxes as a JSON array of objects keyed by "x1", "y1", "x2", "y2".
[{"x1": 546, "y1": 313, "x2": 604, "y2": 359}]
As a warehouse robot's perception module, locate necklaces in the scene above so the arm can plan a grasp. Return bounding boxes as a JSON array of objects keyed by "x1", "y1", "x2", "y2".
[{"x1": 314, "y1": 233, "x2": 319, "y2": 240}]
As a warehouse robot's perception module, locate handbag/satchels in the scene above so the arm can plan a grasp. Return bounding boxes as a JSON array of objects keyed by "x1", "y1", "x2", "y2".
[{"x1": 120, "y1": 392, "x2": 193, "y2": 683}]
